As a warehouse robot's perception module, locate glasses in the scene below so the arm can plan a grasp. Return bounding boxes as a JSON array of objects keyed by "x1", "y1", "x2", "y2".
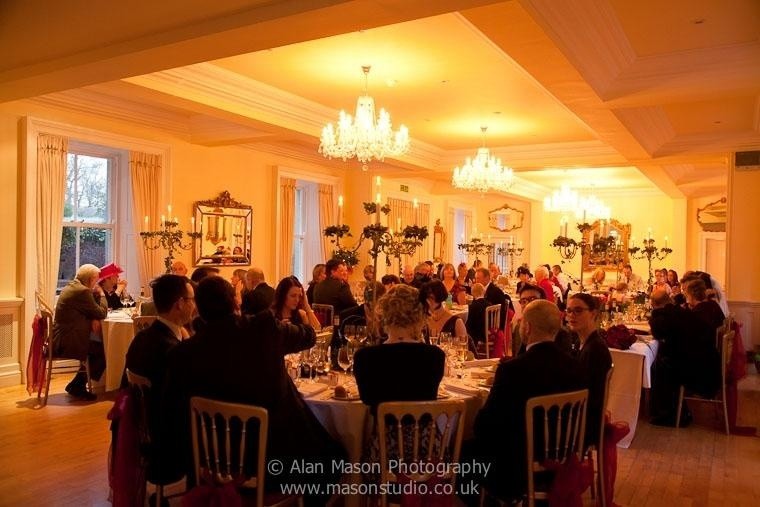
[
  {"x1": 565, "y1": 306, "x2": 590, "y2": 315},
  {"x1": 519, "y1": 295, "x2": 536, "y2": 304},
  {"x1": 184, "y1": 296, "x2": 195, "y2": 302}
]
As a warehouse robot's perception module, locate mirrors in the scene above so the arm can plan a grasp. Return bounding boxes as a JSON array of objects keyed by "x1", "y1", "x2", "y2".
[
  {"x1": 192, "y1": 189, "x2": 252, "y2": 268},
  {"x1": 488, "y1": 204, "x2": 525, "y2": 232},
  {"x1": 578, "y1": 218, "x2": 632, "y2": 287}
]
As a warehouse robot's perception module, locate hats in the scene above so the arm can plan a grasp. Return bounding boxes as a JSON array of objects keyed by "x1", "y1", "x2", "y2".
[{"x1": 98, "y1": 262, "x2": 124, "y2": 280}]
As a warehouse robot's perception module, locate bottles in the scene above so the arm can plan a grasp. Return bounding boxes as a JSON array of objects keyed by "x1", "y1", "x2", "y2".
[
  {"x1": 604, "y1": 305, "x2": 612, "y2": 321},
  {"x1": 611, "y1": 300, "x2": 617, "y2": 315},
  {"x1": 139, "y1": 287, "x2": 145, "y2": 310},
  {"x1": 446, "y1": 293, "x2": 452, "y2": 310},
  {"x1": 331, "y1": 316, "x2": 343, "y2": 370}
]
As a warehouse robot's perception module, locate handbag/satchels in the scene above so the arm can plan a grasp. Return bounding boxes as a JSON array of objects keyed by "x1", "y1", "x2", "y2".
[{"x1": 606, "y1": 324, "x2": 638, "y2": 350}]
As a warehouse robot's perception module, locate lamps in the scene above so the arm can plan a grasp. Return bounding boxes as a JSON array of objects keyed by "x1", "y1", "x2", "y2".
[
  {"x1": 542, "y1": 179, "x2": 612, "y2": 227},
  {"x1": 452, "y1": 124, "x2": 513, "y2": 190},
  {"x1": 318, "y1": 61, "x2": 411, "y2": 161}
]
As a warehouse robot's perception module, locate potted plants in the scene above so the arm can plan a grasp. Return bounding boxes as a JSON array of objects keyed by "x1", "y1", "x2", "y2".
[{"x1": 745, "y1": 345, "x2": 760, "y2": 376}]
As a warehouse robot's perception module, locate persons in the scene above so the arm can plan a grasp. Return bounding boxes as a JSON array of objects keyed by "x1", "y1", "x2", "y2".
[
  {"x1": 232, "y1": 247, "x2": 247, "y2": 263},
  {"x1": 51, "y1": 262, "x2": 727, "y2": 506}
]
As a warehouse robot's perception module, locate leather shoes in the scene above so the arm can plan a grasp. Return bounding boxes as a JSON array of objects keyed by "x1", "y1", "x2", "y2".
[
  {"x1": 69, "y1": 385, "x2": 98, "y2": 400},
  {"x1": 65, "y1": 382, "x2": 71, "y2": 392}
]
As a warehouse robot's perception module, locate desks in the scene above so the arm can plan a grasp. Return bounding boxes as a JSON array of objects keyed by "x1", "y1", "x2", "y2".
[{"x1": 735, "y1": 374, "x2": 760, "y2": 437}]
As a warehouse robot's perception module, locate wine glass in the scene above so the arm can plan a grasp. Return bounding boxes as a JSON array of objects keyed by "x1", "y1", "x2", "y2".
[
  {"x1": 347, "y1": 342, "x2": 360, "y2": 385},
  {"x1": 356, "y1": 326, "x2": 367, "y2": 343},
  {"x1": 287, "y1": 347, "x2": 328, "y2": 384},
  {"x1": 127, "y1": 294, "x2": 135, "y2": 311},
  {"x1": 429, "y1": 332, "x2": 468, "y2": 367},
  {"x1": 338, "y1": 347, "x2": 352, "y2": 388},
  {"x1": 120, "y1": 294, "x2": 128, "y2": 313},
  {"x1": 344, "y1": 325, "x2": 355, "y2": 341}
]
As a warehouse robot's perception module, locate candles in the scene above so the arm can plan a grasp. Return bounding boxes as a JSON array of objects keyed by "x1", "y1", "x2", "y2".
[
  {"x1": 336, "y1": 175, "x2": 418, "y2": 238},
  {"x1": 461, "y1": 226, "x2": 523, "y2": 249},
  {"x1": 631, "y1": 228, "x2": 667, "y2": 249},
  {"x1": 236, "y1": 224, "x2": 240, "y2": 235},
  {"x1": 141, "y1": 204, "x2": 204, "y2": 233}
]
